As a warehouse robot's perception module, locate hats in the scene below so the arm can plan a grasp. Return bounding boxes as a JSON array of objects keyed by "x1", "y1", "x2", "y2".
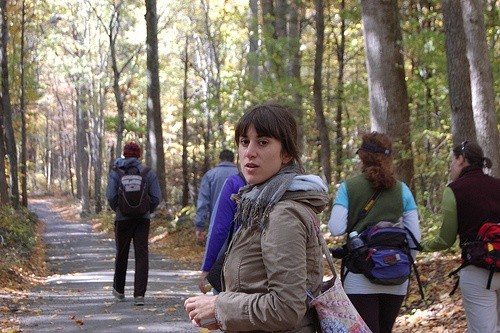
[
  {"x1": 355, "y1": 142, "x2": 391, "y2": 156},
  {"x1": 123, "y1": 141, "x2": 140, "y2": 160}
]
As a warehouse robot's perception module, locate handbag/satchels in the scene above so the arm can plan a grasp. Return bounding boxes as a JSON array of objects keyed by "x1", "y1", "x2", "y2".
[
  {"x1": 292, "y1": 200, "x2": 372, "y2": 333},
  {"x1": 460, "y1": 222, "x2": 500, "y2": 272},
  {"x1": 207, "y1": 205, "x2": 236, "y2": 294}
]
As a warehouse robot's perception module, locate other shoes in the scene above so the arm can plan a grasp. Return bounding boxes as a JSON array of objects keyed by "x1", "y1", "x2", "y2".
[
  {"x1": 134, "y1": 296, "x2": 144, "y2": 305},
  {"x1": 112, "y1": 289, "x2": 125, "y2": 301}
]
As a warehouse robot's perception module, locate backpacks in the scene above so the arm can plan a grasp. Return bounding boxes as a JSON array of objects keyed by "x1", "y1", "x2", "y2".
[
  {"x1": 342, "y1": 220, "x2": 410, "y2": 286},
  {"x1": 111, "y1": 165, "x2": 151, "y2": 217}
]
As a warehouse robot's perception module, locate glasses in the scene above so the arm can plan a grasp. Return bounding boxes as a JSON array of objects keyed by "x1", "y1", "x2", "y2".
[{"x1": 462, "y1": 140, "x2": 470, "y2": 159}]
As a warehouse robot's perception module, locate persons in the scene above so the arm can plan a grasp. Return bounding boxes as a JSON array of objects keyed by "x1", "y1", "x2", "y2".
[
  {"x1": 420, "y1": 140, "x2": 500, "y2": 333},
  {"x1": 328, "y1": 131, "x2": 421, "y2": 333},
  {"x1": 195, "y1": 149, "x2": 240, "y2": 244},
  {"x1": 199, "y1": 172, "x2": 246, "y2": 294},
  {"x1": 106, "y1": 141, "x2": 162, "y2": 306},
  {"x1": 184, "y1": 104, "x2": 333, "y2": 333}
]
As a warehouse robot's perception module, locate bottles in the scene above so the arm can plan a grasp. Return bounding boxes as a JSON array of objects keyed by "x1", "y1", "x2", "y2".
[{"x1": 347, "y1": 230, "x2": 364, "y2": 250}]
